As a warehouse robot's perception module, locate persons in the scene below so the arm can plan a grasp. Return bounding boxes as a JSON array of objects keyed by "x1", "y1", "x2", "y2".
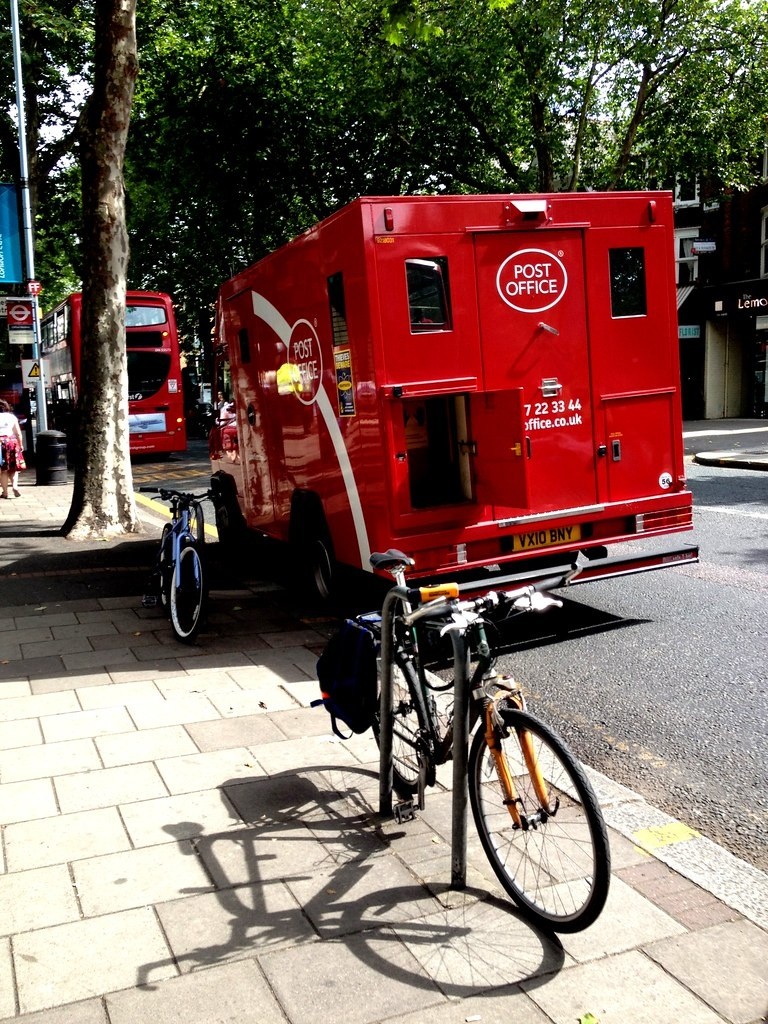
[
  {"x1": 0, "y1": 400, "x2": 26, "y2": 500},
  {"x1": 214, "y1": 391, "x2": 236, "y2": 427}
]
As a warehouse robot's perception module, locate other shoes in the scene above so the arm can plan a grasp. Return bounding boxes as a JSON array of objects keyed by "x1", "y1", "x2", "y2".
[
  {"x1": 0, "y1": 493, "x2": 8, "y2": 498},
  {"x1": 13, "y1": 488, "x2": 21, "y2": 498}
]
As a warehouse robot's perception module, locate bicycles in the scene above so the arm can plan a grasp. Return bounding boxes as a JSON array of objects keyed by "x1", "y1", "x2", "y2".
[
  {"x1": 353, "y1": 550, "x2": 613, "y2": 933},
  {"x1": 137, "y1": 485, "x2": 241, "y2": 643}
]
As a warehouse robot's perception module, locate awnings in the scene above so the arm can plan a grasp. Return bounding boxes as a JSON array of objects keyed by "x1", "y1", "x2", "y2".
[{"x1": 676, "y1": 286, "x2": 694, "y2": 310}]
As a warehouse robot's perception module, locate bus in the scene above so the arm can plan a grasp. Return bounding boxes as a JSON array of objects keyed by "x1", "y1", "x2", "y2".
[{"x1": 40, "y1": 290, "x2": 187, "y2": 460}]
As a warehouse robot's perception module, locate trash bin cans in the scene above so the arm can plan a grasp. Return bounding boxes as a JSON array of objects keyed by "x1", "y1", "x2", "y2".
[
  {"x1": 200, "y1": 383, "x2": 211, "y2": 403},
  {"x1": 35, "y1": 430, "x2": 68, "y2": 486},
  {"x1": 199, "y1": 403, "x2": 214, "y2": 413}
]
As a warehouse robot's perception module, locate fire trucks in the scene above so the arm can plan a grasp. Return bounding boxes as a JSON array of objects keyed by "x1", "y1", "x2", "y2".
[{"x1": 207, "y1": 190, "x2": 700, "y2": 617}]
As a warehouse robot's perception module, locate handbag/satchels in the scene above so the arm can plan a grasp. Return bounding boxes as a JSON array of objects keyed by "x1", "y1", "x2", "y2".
[{"x1": 315, "y1": 624, "x2": 377, "y2": 739}]
